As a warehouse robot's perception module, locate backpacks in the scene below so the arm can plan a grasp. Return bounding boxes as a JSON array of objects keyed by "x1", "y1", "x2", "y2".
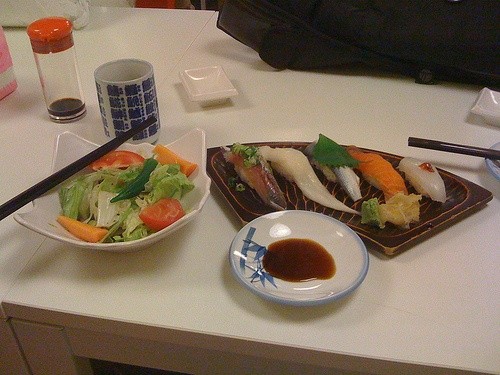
[{"x1": 217, "y1": 0, "x2": 500, "y2": 92}]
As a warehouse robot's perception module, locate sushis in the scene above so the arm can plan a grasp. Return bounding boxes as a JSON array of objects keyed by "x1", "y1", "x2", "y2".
[{"x1": 221, "y1": 141, "x2": 447, "y2": 216}]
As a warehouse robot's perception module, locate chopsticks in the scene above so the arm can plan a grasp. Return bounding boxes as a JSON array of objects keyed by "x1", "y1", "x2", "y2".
[
  {"x1": 407, "y1": 136, "x2": 499, "y2": 163},
  {"x1": 0, "y1": 110, "x2": 157, "y2": 222}
]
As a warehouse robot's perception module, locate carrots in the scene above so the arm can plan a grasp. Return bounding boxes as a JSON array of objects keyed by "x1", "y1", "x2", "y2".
[
  {"x1": 152, "y1": 144, "x2": 198, "y2": 177},
  {"x1": 56, "y1": 215, "x2": 109, "y2": 243}
]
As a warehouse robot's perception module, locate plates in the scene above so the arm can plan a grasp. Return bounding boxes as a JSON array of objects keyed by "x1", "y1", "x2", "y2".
[
  {"x1": 13, "y1": 130, "x2": 212, "y2": 251},
  {"x1": 204, "y1": 142, "x2": 492, "y2": 257},
  {"x1": 230, "y1": 211, "x2": 369, "y2": 307},
  {"x1": 180, "y1": 65, "x2": 238, "y2": 108},
  {"x1": 485, "y1": 141, "x2": 499, "y2": 181}
]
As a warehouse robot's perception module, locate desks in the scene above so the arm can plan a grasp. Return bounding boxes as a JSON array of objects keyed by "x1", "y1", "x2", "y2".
[{"x1": 0, "y1": 5, "x2": 500, "y2": 375}]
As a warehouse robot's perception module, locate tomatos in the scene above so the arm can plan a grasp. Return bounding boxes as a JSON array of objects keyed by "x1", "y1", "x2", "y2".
[
  {"x1": 139, "y1": 197, "x2": 185, "y2": 231},
  {"x1": 91, "y1": 150, "x2": 145, "y2": 171}
]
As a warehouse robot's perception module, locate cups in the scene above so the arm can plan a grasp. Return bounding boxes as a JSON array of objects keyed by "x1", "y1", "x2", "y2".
[{"x1": 92, "y1": 59, "x2": 160, "y2": 146}]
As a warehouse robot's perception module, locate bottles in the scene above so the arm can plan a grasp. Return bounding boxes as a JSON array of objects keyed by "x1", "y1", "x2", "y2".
[{"x1": 27, "y1": 17, "x2": 86, "y2": 123}]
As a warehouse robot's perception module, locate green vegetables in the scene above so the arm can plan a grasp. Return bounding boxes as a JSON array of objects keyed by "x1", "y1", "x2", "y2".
[
  {"x1": 58, "y1": 157, "x2": 195, "y2": 243},
  {"x1": 312, "y1": 134, "x2": 360, "y2": 168}
]
